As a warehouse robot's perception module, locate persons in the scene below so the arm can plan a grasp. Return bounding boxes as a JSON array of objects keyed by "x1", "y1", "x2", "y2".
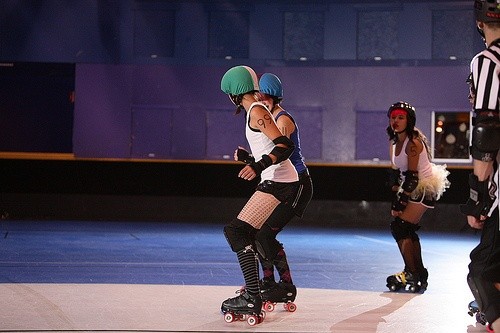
[
  {"x1": 234, "y1": 72, "x2": 313, "y2": 314},
  {"x1": 465, "y1": 0, "x2": 500, "y2": 332},
  {"x1": 219, "y1": 65, "x2": 300, "y2": 324},
  {"x1": 384, "y1": 102, "x2": 450, "y2": 294}
]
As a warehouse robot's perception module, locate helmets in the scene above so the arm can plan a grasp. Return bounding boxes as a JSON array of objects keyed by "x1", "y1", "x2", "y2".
[
  {"x1": 387, "y1": 101, "x2": 416, "y2": 123},
  {"x1": 257, "y1": 72, "x2": 283, "y2": 96},
  {"x1": 474, "y1": 0, "x2": 500, "y2": 20},
  {"x1": 221, "y1": 65, "x2": 260, "y2": 94}
]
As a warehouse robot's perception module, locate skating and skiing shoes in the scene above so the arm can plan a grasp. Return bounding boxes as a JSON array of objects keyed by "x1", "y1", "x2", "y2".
[
  {"x1": 468, "y1": 300, "x2": 488, "y2": 325},
  {"x1": 262, "y1": 278, "x2": 297, "y2": 312},
  {"x1": 221, "y1": 291, "x2": 266, "y2": 325},
  {"x1": 386, "y1": 266, "x2": 429, "y2": 293},
  {"x1": 239, "y1": 276, "x2": 276, "y2": 294}
]
